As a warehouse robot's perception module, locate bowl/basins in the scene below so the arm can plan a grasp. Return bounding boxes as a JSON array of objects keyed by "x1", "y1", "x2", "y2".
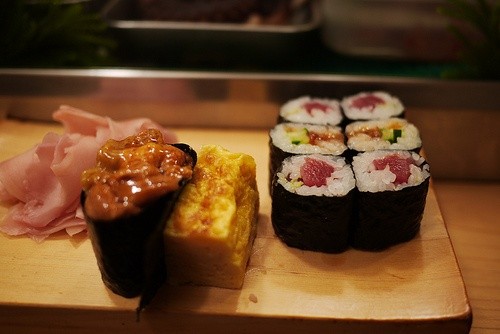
[{"x1": 111, "y1": 0, "x2": 321, "y2": 68}]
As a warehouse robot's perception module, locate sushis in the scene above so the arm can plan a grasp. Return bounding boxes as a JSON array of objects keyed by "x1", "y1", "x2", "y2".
[{"x1": 268, "y1": 90, "x2": 433, "y2": 252}]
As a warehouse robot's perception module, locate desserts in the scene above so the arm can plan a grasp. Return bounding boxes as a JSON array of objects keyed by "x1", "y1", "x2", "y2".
[{"x1": 163, "y1": 145, "x2": 259, "y2": 288}]
